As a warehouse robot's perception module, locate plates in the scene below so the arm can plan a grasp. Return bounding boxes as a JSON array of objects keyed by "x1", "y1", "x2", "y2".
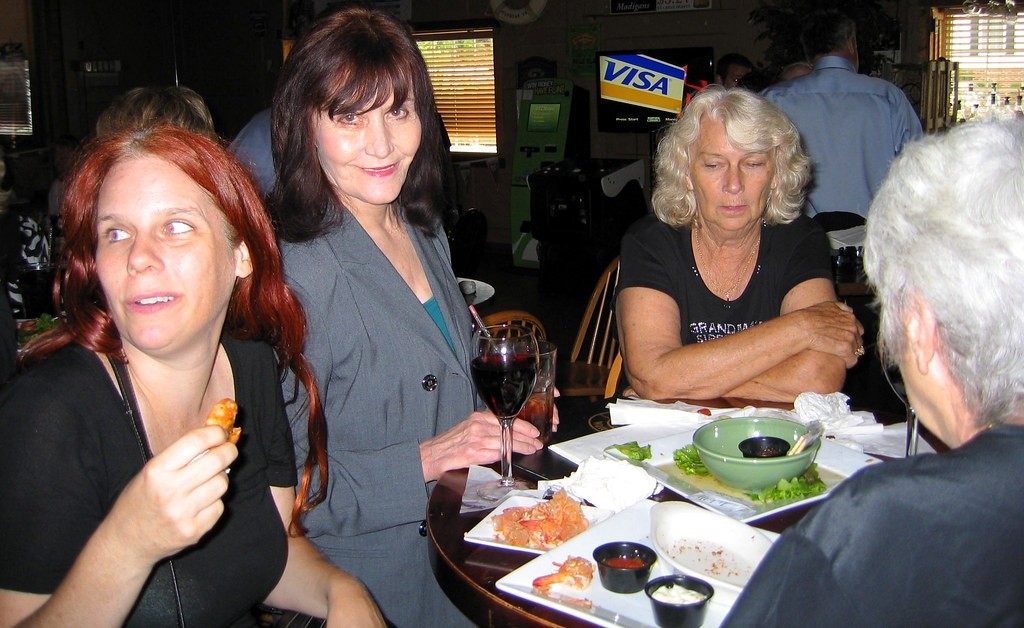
[
  {"x1": 647, "y1": 500, "x2": 774, "y2": 593},
  {"x1": 14, "y1": 318, "x2": 56, "y2": 352},
  {"x1": 464, "y1": 496, "x2": 615, "y2": 558},
  {"x1": 493, "y1": 499, "x2": 784, "y2": 628},
  {"x1": 604, "y1": 420, "x2": 884, "y2": 522}
]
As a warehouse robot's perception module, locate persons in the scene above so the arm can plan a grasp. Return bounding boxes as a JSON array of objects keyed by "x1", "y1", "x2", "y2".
[
  {"x1": 0, "y1": 118, "x2": 389, "y2": 628},
  {"x1": 734, "y1": 70, "x2": 780, "y2": 93},
  {"x1": 718, "y1": 112, "x2": 1024, "y2": 628},
  {"x1": 779, "y1": 61, "x2": 814, "y2": 81},
  {"x1": 0, "y1": 83, "x2": 216, "y2": 321},
  {"x1": 717, "y1": 52, "x2": 756, "y2": 89},
  {"x1": 262, "y1": 7, "x2": 560, "y2": 628},
  {"x1": 758, "y1": 6, "x2": 925, "y2": 425},
  {"x1": 612, "y1": 81, "x2": 864, "y2": 403}
]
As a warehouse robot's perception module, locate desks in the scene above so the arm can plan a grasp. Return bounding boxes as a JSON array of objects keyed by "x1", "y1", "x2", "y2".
[{"x1": 428, "y1": 397, "x2": 907, "y2": 628}]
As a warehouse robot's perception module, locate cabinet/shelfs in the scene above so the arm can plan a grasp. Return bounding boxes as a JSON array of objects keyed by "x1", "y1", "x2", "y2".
[
  {"x1": 885, "y1": 57, "x2": 959, "y2": 134},
  {"x1": 528, "y1": 157, "x2": 646, "y2": 296}
]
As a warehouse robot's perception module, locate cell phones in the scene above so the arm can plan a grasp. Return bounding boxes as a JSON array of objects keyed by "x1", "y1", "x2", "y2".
[{"x1": 510, "y1": 448, "x2": 579, "y2": 484}]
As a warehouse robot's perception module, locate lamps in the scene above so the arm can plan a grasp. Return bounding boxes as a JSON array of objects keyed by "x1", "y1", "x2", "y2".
[{"x1": 961, "y1": 0, "x2": 1018, "y2": 24}]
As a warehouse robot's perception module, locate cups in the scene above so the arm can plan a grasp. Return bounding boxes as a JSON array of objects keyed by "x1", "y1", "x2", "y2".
[{"x1": 500, "y1": 341, "x2": 557, "y2": 447}]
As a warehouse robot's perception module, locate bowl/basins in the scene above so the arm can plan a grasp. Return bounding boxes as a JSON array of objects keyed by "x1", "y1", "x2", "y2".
[
  {"x1": 692, "y1": 418, "x2": 820, "y2": 491},
  {"x1": 644, "y1": 573, "x2": 715, "y2": 628},
  {"x1": 592, "y1": 541, "x2": 655, "y2": 594}
]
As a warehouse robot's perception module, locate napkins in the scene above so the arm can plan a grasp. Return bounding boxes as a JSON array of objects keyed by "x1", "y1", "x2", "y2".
[{"x1": 547, "y1": 396, "x2": 937, "y2": 515}]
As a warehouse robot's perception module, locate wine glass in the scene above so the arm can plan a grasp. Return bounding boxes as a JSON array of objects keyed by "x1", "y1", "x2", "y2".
[{"x1": 470, "y1": 324, "x2": 542, "y2": 498}]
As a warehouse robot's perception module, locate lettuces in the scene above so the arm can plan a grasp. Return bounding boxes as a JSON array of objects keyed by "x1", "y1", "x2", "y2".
[
  {"x1": 749, "y1": 463, "x2": 827, "y2": 501},
  {"x1": 602, "y1": 441, "x2": 708, "y2": 477},
  {"x1": 15, "y1": 314, "x2": 60, "y2": 348}
]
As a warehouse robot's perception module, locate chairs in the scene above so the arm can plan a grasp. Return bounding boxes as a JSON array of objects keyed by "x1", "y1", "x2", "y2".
[{"x1": 476, "y1": 257, "x2": 622, "y2": 399}]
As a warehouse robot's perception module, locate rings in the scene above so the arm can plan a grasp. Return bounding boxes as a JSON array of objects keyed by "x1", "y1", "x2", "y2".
[{"x1": 854, "y1": 345, "x2": 864, "y2": 355}]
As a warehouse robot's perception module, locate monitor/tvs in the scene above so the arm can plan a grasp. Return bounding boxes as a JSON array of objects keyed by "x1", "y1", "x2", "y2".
[
  {"x1": 528, "y1": 104, "x2": 560, "y2": 133},
  {"x1": 595, "y1": 47, "x2": 714, "y2": 134}
]
